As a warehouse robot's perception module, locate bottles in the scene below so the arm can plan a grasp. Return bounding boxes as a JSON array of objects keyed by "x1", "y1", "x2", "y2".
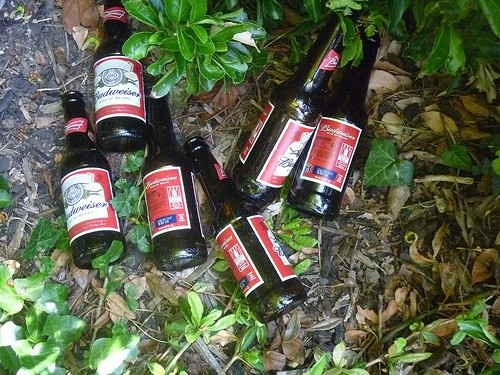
[
  {"x1": 184, "y1": 136, "x2": 307, "y2": 323},
  {"x1": 144, "y1": 82, "x2": 208, "y2": 270},
  {"x1": 230, "y1": 12, "x2": 358, "y2": 206},
  {"x1": 288, "y1": 28, "x2": 382, "y2": 222},
  {"x1": 60, "y1": 91, "x2": 127, "y2": 269},
  {"x1": 92, "y1": 0, "x2": 151, "y2": 152}
]
